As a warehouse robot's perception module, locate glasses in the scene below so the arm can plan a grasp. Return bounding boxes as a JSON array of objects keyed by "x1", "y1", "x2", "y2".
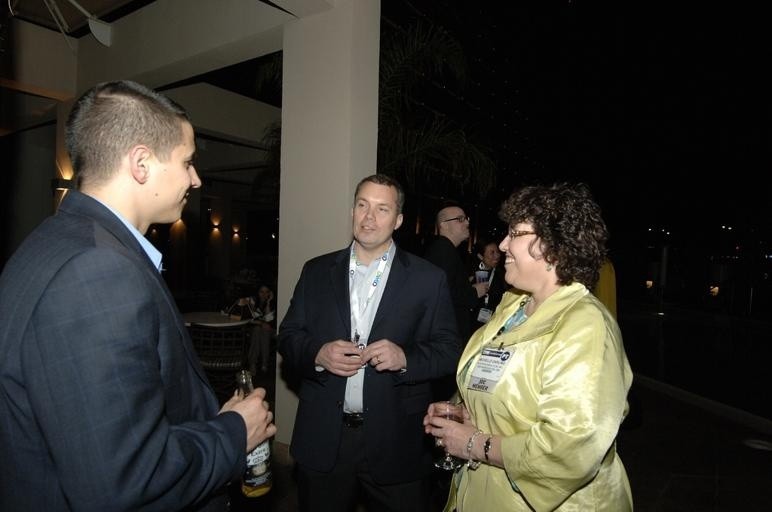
[
  {"x1": 442, "y1": 215, "x2": 469, "y2": 223},
  {"x1": 508, "y1": 225, "x2": 546, "y2": 238}
]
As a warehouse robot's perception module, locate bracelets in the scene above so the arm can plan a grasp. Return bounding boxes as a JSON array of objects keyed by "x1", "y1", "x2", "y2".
[
  {"x1": 483, "y1": 434, "x2": 495, "y2": 467},
  {"x1": 466, "y1": 429, "x2": 483, "y2": 469}
]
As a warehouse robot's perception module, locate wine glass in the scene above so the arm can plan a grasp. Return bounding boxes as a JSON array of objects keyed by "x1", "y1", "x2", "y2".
[{"x1": 432, "y1": 401, "x2": 468, "y2": 470}]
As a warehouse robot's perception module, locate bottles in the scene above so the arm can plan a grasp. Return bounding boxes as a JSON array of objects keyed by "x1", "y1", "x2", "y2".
[{"x1": 232, "y1": 366, "x2": 273, "y2": 499}]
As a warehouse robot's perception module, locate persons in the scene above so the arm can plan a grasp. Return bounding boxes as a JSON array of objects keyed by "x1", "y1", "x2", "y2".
[
  {"x1": 274, "y1": 174, "x2": 465, "y2": 511},
  {"x1": 421, "y1": 203, "x2": 489, "y2": 339},
  {"x1": 470, "y1": 234, "x2": 510, "y2": 331},
  {"x1": 0, "y1": 79, "x2": 278, "y2": 510},
  {"x1": 420, "y1": 177, "x2": 637, "y2": 511},
  {"x1": 230, "y1": 284, "x2": 274, "y2": 377}
]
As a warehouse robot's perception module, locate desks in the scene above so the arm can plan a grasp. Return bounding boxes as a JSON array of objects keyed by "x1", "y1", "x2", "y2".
[{"x1": 181, "y1": 313, "x2": 253, "y2": 327}]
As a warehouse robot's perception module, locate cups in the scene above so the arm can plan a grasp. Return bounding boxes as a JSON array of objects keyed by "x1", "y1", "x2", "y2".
[{"x1": 475, "y1": 270, "x2": 489, "y2": 284}]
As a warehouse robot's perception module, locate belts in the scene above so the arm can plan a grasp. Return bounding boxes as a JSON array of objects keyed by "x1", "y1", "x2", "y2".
[{"x1": 344, "y1": 414, "x2": 363, "y2": 429}]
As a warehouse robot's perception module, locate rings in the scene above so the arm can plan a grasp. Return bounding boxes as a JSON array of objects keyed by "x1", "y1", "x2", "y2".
[
  {"x1": 433, "y1": 436, "x2": 443, "y2": 448},
  {"x1": 376, "y1": 354, "x2": 381, "y2": 364}
]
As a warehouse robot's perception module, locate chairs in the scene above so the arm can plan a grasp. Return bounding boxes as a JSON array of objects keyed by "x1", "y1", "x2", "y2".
[{"x1": 191, "y1": 322, "x2": 248, "y2": 385}]
